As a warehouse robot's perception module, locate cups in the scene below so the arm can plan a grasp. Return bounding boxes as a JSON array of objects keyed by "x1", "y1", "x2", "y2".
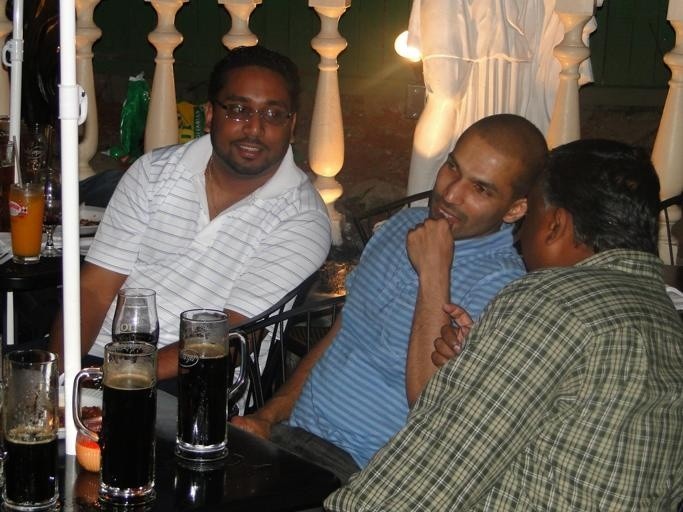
[
  {"x1": 9, "y1": 183, "x2": 45, "y2": 263},
  {"x1": 5, "y1": 347, "x2": 61, "y2": 511},
  {"x1": 176, "y1": 308, "x2": 247, "y2": 462},
  {"x1": 73, "y1": 341, "x2": 159, "y2": 507},
  {"x1": 111, "y1": 288, "x2": 160, "y2": 356}
]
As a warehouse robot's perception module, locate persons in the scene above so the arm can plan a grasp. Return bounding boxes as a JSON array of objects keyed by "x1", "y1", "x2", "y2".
[
  {"x1": 228, "y1": 113, "x2": 549, "y2": 495},
  {"x1": 321, "y1": 132, "x2": 683, "y2": 511},
  {"x1": 0, "y1": 43, "x2": 335, "y2": 425}
]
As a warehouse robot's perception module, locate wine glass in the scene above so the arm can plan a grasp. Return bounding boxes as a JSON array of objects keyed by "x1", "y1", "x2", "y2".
[{"x1": 43, "y1": 200, "x2": 62, "y2": 253}]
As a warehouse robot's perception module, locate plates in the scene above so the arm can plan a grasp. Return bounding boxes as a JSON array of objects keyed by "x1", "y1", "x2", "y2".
[
  {"x1": 53, "y1": 204, "x2": 107, "y2": 255},
  {"x1": 56, "y1": 384, "x2": 104, "y2": 441}
]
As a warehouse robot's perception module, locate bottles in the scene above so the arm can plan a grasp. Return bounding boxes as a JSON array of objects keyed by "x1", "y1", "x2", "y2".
[
  {"x1": 44, "y1": 169, "x2": 57, "y2": 211},
  {"x1": 31, "y1": 124, "x2": 45, "y2": 177}
]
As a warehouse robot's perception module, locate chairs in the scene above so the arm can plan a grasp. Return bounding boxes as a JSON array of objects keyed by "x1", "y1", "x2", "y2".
[
  {"x1": 212, "y1": 263, "x2": 325, "y2": 414},
  {"x1": 650, "y1": 191, "x2": 683, "y2": 318},
  {"x1": 356, "y1": 184, "x2": 436, "y2": 242},
  {"x1": 229, "y1": 294, "x2": 351, "y2": 438}
]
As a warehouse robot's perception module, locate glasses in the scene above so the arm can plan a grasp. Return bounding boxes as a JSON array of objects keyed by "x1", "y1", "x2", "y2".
[{"x1": 209, "y1": 94, "x2": 299, "y2": 127}]
